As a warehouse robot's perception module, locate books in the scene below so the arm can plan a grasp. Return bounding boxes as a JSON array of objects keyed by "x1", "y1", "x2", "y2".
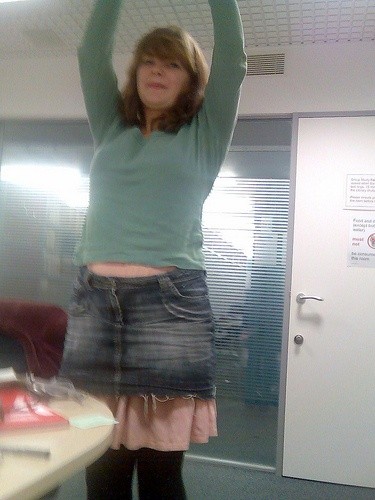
[{"x1": 0, "y1": 384, "x2": 70, "y2": 435}]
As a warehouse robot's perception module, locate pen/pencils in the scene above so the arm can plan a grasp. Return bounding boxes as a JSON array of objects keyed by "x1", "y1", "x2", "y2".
[{"x1": 0, "y1": 445, "x2": 52, "y2": 460}]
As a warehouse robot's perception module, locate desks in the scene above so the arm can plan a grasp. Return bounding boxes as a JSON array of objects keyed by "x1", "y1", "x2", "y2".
[{"x1": 2, "y1": 371, "x2": 118, "y2": 500}]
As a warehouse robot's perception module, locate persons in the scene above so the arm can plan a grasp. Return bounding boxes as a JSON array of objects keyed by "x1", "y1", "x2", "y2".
[
  {"x1": 238, "y1": 256, "x2": 284, "y2": 408},
  {"x1": 57, "y1": 1, "x2": 249, "y2": 499}
]
